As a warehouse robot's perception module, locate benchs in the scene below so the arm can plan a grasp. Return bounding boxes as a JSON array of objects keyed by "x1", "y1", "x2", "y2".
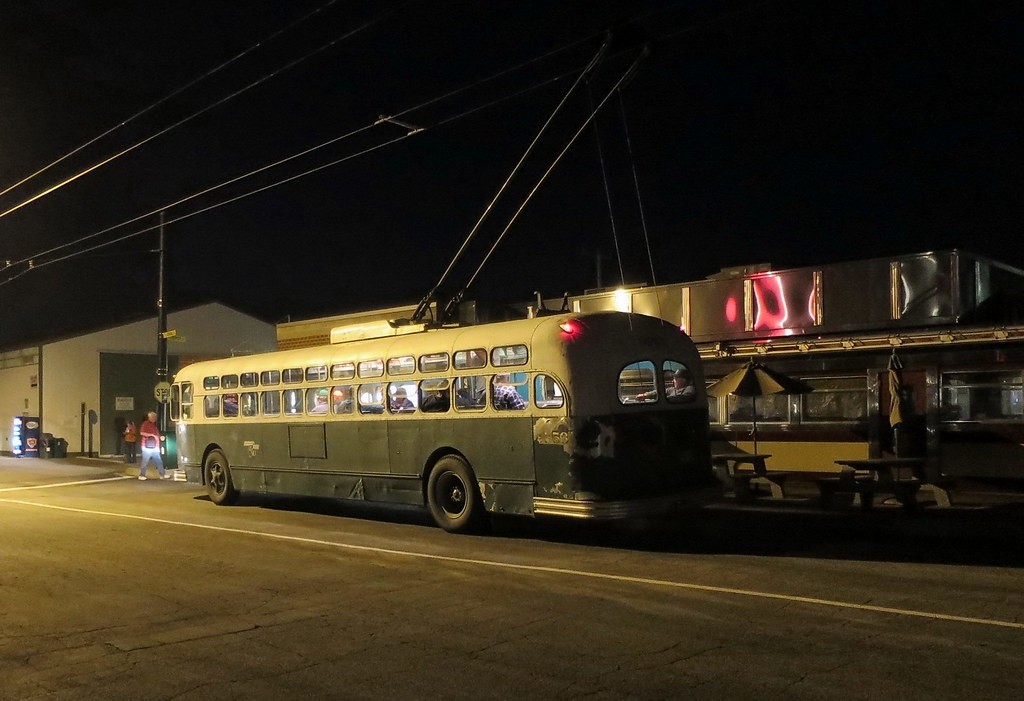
[
  {"x1": 813, "y1": 473, "x2": 959, "y2": 507},
  {"x1": 711, "y1": 471, "x2": 789, "y2": 505}
]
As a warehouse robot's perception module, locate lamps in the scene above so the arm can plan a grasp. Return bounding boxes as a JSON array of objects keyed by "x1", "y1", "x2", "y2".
[
  {"x1": 755, "y1": 344, "x2": 769, "y2": 354},
  {"x1": 992, "y1": 326, "x2": 1009, "y2": 339},
  {"x1": 797, "y1": 342, "x2": 810, "y2": 351},
  {"x1": 939, "y1": 330, "x2": 955, "y2": 341},
  {"x1": 887, "y1": 335, "x2": 903, "y2": 345},
  {"x1": 842, "y1": 338, "x2": 855, "y2": 348}
]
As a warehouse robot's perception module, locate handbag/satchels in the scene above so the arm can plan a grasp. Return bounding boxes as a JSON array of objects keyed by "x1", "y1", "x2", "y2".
[{"x1": 146, "y1": 439, "x2": 156, "y2": 448}]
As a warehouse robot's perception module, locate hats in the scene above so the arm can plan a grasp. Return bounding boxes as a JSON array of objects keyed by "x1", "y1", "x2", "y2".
[
  {"x1": 320, "y1": 389, "x2": 327, "y2": 396},
  {"x1": 393, "y1": 388, "x2": 408, "y2": 399},
  {"x1": 672, "y1": 369, "x2": 689, "y2": 378}
]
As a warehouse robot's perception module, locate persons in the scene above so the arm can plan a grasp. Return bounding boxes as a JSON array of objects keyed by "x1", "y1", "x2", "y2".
[
  {"x1": 391, "y1": 388, "x2": 414, "y2": 407},
  {"x1": 310, "y1": 386, "x2": 362, "y2": 413},
  {"x1": 477, "y1": 374, "x2": 526, "y2": 410},
  {"x1": 423, "y1": 381, "x2": 448, "y2": 410},
  {"x1": 224, "y1": 395, "x2": 237, "y2": 415},
  {"x1": 138, "y1": 412, "x2": 171, "y2": 480},
  {"x1": 124, "y1": 420, "x2": 138, "y2": 463},
  {"x1": 636, "y1": 368, "x2": 694, "y2": 401}
]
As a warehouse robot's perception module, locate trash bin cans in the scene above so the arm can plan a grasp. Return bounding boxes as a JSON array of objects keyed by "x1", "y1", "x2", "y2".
[
  {"x1": 54, "y1": 437, "x2": 69, "y2": 457},
  {"x1": 38, "y1": 433, "x2": 54, "y2": 459},
  {"x1": 159, "y1": 431, "x2": 177, "y2": 469}
]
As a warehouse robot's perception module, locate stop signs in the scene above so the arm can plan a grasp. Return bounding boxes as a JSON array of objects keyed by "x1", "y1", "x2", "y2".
[{"x1": 154, "y1": 381, "x2": 173, "y2": 404}]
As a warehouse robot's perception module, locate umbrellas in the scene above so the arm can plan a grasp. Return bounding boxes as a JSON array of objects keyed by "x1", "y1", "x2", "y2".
[
  {"x1": 887, "y1": 354, "x2": 904, "y2": 481},
  {"x1": 706, "y1": 357, "x2": 815, "y2": 454}
]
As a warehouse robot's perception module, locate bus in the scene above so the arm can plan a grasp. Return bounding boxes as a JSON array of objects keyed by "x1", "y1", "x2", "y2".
[
  {"x1": 620, "y1": 322, "x2": 1024, "y2": 510},
  {"x1": 169, "y1": 309, "x2": 726, "y2": 536}
]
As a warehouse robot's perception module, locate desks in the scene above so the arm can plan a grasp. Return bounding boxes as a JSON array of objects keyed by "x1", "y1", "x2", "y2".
[
  {"x1": 711, "y1": 452, "x2": 772, "y2": 503},
  {"x1": 836, "y1": 458, "x2": 931, "y2": 511}
]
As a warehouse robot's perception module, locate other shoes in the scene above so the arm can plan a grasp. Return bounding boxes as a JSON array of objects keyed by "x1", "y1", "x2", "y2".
[
  {"x1": 160, "y1": 474, "x2": 171, "y2": 480},
  {"x1": 138, "y1": 476, "x2": 148, "y2": 481}
]
